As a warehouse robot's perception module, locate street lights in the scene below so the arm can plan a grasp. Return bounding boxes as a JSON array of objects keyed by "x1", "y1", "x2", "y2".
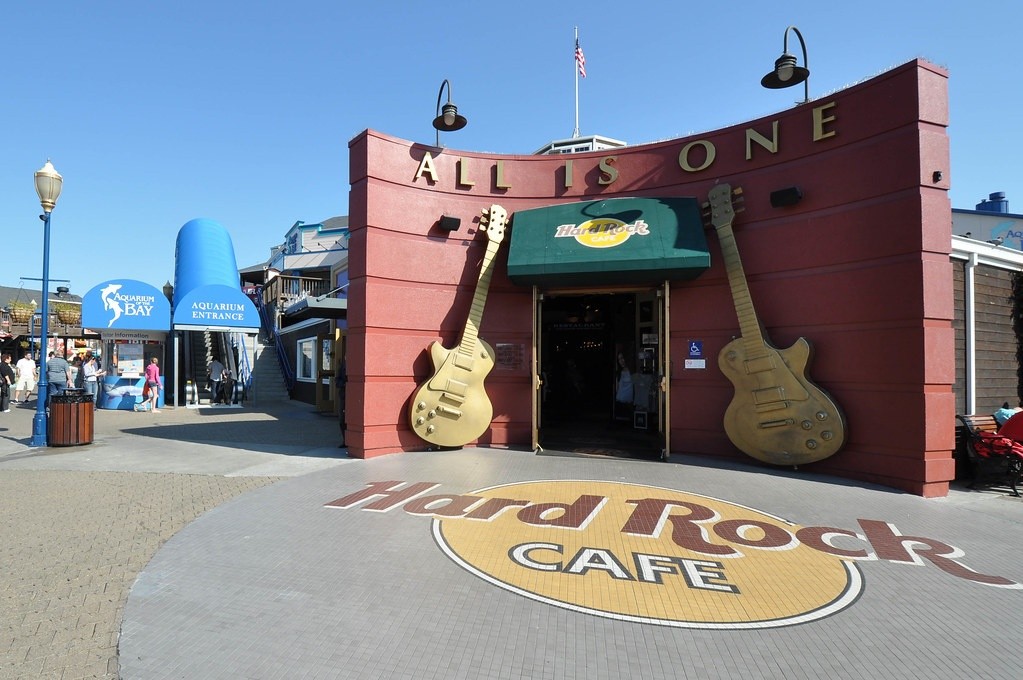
[{"x1": 27, "y1": 160, "x2": 64, "y2": 448}]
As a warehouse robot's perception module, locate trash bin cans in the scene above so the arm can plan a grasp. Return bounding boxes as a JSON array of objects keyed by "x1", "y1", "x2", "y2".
[{"x1": 50, "y1": 386, "x2": 96, "y2": 446}]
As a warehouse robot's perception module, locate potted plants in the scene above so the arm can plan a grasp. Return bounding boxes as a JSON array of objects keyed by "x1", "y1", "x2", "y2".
[
  {"x1": 55, "y1": 302, "x2": 81, "y2": 324},
  {"x1": 7, "y1": 298, "x2": 37, "y2": 323}
]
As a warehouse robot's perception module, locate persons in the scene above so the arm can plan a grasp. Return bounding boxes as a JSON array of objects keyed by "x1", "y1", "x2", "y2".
[
  {"x1": 73, "y1": 354, "x2": 81, "y2": 362},
  {"x1": 10, "y1": 351, "x2": 36, "y2": 405},
  {"x1": 0, "y1": 354, "x2": 14, "y2": 413},
  {"x1": 81, "y1": 353, "x2": 85, "y2": 360},
  {"x1": 992, "y1": 409, "x2": 1023, "y2": 467},
  {"x1": 615, "y1": 352, "x2": 633, "y2": 411},
  {"x1": 134, "y1": 357, "x2": 163, "y2": 414},
  {"x1": 207, "y1": 356, "x2": 227, "y2": 404},
  {"x1": 46, "y1": 350, "x2": 71, "y2": 408},
  {"x1": 82, "y1": 351, "x2": 106, "y2": 412}
]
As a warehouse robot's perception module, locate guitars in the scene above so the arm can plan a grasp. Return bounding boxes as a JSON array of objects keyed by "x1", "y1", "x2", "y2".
[
  {"x1": 406, "y1": 204, "x2": 510, "y2": 449},
  {"x1": 702, "y1": 180, "x2": 850, "y2": 469}
]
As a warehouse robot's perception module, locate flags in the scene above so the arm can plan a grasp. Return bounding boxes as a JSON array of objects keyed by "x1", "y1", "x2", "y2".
[{"x1": 575, "y1": 37, "x2": 586, "y2": 79}]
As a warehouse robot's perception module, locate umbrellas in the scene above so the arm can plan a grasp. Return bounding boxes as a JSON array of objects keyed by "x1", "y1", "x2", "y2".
[{"x1": 79, "y1": 348, "x2": 86, "y2": 354}]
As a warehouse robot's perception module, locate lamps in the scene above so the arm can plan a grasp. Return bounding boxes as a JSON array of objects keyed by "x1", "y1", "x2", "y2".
[
  {"x1": 301, "y1": 245, "x2": 311, "y2": 252},
  {"x1": 761, "y1": 25, "x2": 810, "y2": 106},
  {"x1": 432, "y1": 78, "x2": 468, "y2": 147},
  {"x1": 318, "y1": 242, "x2": 328, "y2": 250},
  {"x1": 335, "y1": 241, "x2": 345, "y2": 249}
]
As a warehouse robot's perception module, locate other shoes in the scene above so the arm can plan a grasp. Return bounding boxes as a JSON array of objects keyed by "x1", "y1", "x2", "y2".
[
  {"x1": 151, "y1": 410, "x2": 161, "y2": 413},
  {"x1": 9, "y1": 399, "x2": 18, "y2": 404},
  {"x1": 25, "y1": 401, "x2": 31, "y2": 404},
  {"x1": 141, "y1": 403, "x2": 146, "y2": 410},
  {"x1": 4, "y1": 409, "x2": 10, "y2": 413}
]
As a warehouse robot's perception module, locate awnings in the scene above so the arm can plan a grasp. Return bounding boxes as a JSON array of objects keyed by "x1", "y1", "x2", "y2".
[{"x1": 507, "y1": 196, "x2": 711, "y2": 287}]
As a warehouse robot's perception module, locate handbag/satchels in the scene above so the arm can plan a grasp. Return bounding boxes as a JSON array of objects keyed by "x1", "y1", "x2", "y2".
[{"x1": 0, "y1": 373, "x2": 5, "y2": 386}]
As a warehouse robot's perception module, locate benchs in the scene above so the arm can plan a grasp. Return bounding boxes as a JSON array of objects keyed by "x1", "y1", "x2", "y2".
[{"x1": 955, "y1": 414, "x2": 1023, "y2": 486}]
